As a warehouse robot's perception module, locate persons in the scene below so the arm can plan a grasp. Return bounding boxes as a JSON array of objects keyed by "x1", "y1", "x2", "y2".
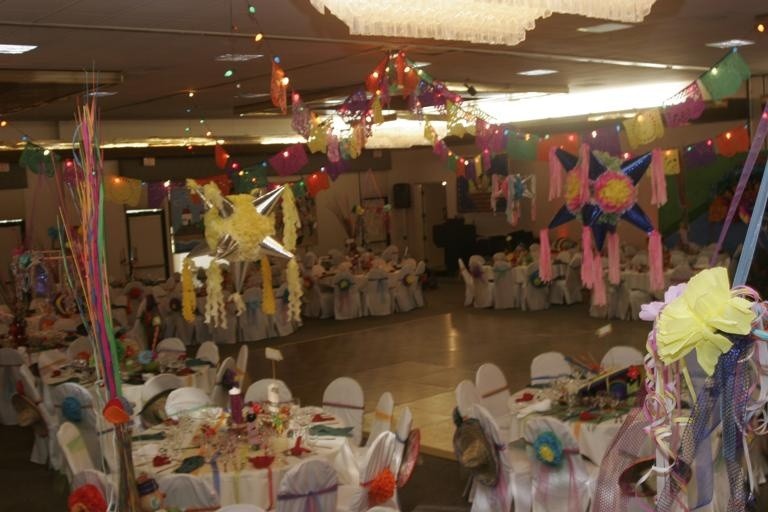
[{"x1": 704, "y1": 165, "x2": 768, "y2": 303}]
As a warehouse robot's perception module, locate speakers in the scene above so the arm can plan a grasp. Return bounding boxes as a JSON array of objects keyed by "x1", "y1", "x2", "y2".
[{"x1": 393, "y1": 183, "x2": 411, "y2": 209}]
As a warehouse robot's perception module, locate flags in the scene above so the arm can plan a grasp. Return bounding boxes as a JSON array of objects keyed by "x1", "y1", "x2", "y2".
[{"x1": 186, "y1": 52, "x2": 623, "y2": 206}]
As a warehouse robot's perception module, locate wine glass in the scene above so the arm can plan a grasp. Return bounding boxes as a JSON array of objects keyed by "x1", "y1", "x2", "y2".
[{"x1": 132, "y1": 397, "x2": 336, "y2": 474}]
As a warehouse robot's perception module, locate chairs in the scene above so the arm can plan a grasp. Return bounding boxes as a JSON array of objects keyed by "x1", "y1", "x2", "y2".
[{"x1": 457, "y1": 242, "x2": 732, "y2": 322}]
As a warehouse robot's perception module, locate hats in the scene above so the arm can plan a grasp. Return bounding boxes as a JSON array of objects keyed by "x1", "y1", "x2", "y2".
[
  {"x1": 576, "y1": 364, "x2": 644, "y2": 400},
  {"x1": 11, "y1": 394, "x2": 49, "y2": 437},
  {"x1": 141, "y1": 388, "x2": 174, "y2": 424},
  {"x1": 454, "y1": 419, "x2": 499, "y2": 485},
  {"x1": 619, "y1": 457, "x2": 692, "y2": 497}
]
